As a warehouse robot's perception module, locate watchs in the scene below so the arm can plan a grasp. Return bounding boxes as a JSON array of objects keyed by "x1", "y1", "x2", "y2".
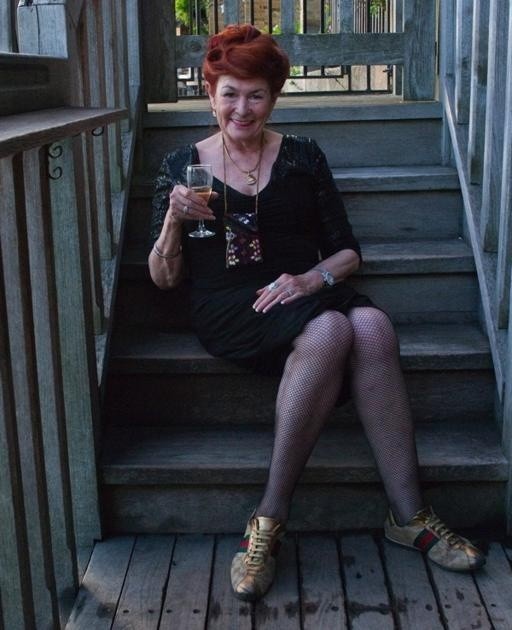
[{"x1": 313, "y1": 266, "x2": 334, "y2": 290}]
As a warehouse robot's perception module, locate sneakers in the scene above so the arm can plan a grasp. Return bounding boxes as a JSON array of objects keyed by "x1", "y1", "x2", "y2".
[
  {"x1": 382, "y1": 507, "x2": 488, "y2": 572},
  {"x1": 228, "y1": 506, "x2": 286, "y2": 600}
]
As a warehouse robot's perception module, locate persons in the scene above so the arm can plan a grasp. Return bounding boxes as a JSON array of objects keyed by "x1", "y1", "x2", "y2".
[{"x1": 147, "y1": 22, "x2": 484, "y2": 602}]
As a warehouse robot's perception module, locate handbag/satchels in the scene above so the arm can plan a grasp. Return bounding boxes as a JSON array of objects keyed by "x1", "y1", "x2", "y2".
[{"x1": 224, "y1": 212, "x2": 265, "y2": 269}]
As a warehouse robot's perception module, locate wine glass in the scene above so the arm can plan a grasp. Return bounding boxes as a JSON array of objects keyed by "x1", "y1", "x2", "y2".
[{"x1": 187, "y1": 164, "x2": 215, "y2": 238}]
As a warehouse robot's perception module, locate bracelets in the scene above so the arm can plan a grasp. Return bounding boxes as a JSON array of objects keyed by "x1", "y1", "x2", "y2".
[{"x1": 152, "y1": 243, "x2": 183, "y2": 259}]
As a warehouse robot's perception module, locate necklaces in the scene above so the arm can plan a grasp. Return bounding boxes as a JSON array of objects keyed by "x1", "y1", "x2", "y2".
[{"x1": 221, "y1": 131, "x2": 263, "y2": 186}]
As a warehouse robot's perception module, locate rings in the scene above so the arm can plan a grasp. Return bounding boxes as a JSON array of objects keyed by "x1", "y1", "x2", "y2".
[
  {"x1": 268, "y1": 281, "x2": 279, "y2": 289},
  {"x1": 183, "y1": 206, "x2": 189, "y2": 215},
  {"x1": 286, "y1": 289, "x2": 293, "y2": 296}
]
